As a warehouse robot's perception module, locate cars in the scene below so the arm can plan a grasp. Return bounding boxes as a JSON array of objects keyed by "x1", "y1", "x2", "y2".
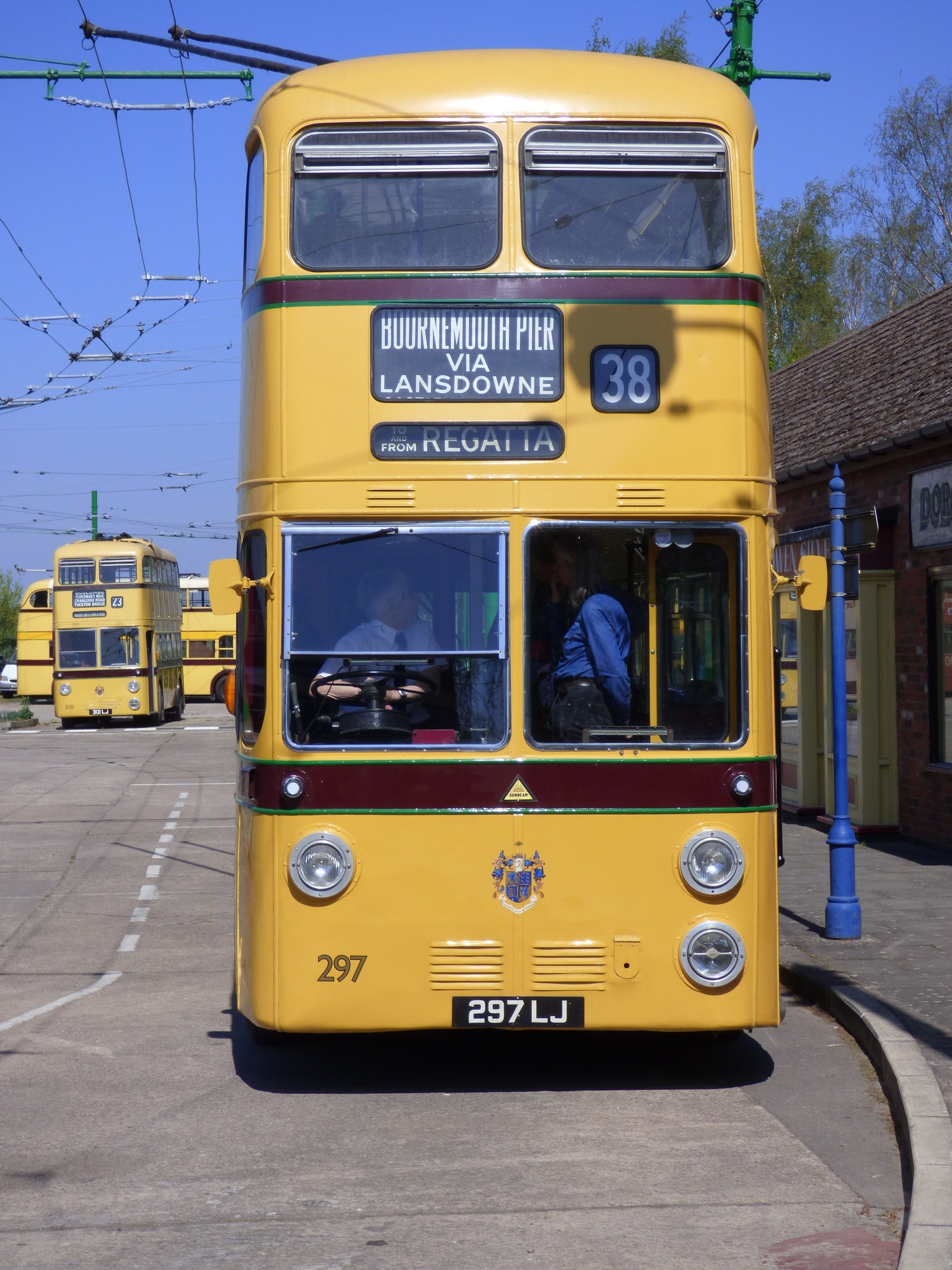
[{"x1": 0, "y1": 646, "x2": 17, "y2": 699}]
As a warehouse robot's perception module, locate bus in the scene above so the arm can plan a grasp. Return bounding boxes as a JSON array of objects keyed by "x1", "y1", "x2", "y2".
[
  {"x1": 207, "y1": 46, "x2": 830, "y2": 1037},
  {"x1": 13, "y1": 532, "x2": 240, "y2": 729}
]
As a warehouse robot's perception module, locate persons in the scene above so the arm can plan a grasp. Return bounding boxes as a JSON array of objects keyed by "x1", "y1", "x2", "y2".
[
  {"x1": 549, "y1": 533, "x2": 649, "y2": 742},
  {"x1": 309, "y1": 565, "x2": 450, "y2": 709}
]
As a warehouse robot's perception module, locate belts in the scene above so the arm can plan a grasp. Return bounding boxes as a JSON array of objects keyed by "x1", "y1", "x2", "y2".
[{"x1": 557, "y1": 679, "x2": 601, "y2": 693}]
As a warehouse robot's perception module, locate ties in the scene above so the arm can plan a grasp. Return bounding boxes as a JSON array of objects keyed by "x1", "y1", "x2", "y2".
[{"x1": 394, "y1": 631, "x2": 407, "y2": 650}]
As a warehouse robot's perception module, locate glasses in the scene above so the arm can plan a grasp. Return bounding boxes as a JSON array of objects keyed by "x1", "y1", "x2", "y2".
[{"x1": 385, "y1": 588, "x2": 418, "y2": 604}]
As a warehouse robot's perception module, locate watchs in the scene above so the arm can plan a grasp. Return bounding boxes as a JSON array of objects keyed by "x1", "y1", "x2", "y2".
[{"x1": 398, "y1": 688, "x2": 407, "y2": 702}]
